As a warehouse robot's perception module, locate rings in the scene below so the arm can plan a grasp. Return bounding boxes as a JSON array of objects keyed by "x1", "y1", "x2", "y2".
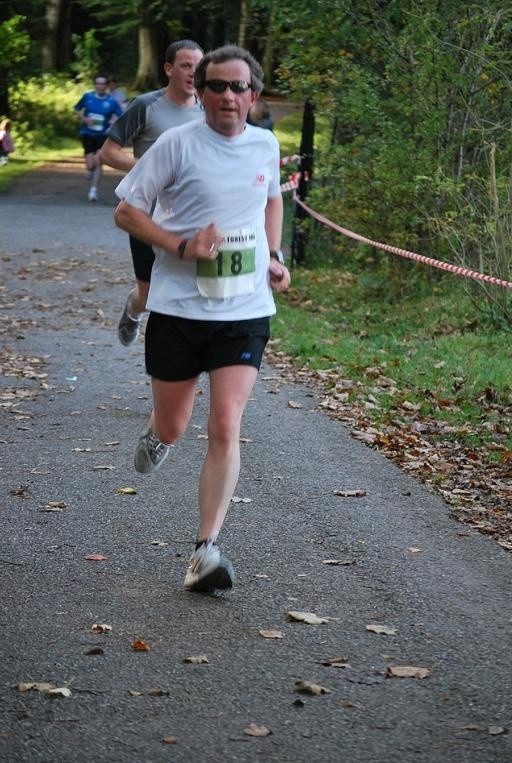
[{"x1": 208, "y1": 243, "x2": 215, "y2": 253}]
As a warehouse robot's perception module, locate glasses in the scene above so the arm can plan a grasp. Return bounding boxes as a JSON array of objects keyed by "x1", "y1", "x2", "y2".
[{"x1": 204, "y1": 78, "x2": 252, "y2": 94}]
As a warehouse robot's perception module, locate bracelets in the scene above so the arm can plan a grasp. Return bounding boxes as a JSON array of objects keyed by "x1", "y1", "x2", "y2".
[
  {"x1": 177, "y1": 240, "x2": 187, "y2": 262},
  {"x1": 270, "y1": 250, "x2": 283, "y2": 264}
]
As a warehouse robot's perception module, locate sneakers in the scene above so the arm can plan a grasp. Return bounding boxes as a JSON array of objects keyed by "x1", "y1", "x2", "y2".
[
  {"x1": 118, "y1": 286, "x2": 143, "y2": 348},
  {"x1": 132, "y1": 406, "x2": 175, "y2": 475},
  {"x1": 182, "y1": 543, "x2": 235, "y2": 595},
  {"x1": 87, "y1": 190, "x2": 99, "y2": 203}
]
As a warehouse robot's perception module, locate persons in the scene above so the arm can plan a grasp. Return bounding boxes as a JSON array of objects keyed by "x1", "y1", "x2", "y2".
[
  {"x1": 72, "y1": 73, "x2": 124, "y2": 203},
  {"x1": 99, "y1": 39, "x2": 207, "y2": 347},
  {"x1": 250, "y1": 100, "x2": 273, "y2": 128},
  {"x1": 111, "y1": 43, "x2": 293, "y2": 594},
  {"x1": 107, "y1": 79, "x2": 125, "y2": 130}
]
